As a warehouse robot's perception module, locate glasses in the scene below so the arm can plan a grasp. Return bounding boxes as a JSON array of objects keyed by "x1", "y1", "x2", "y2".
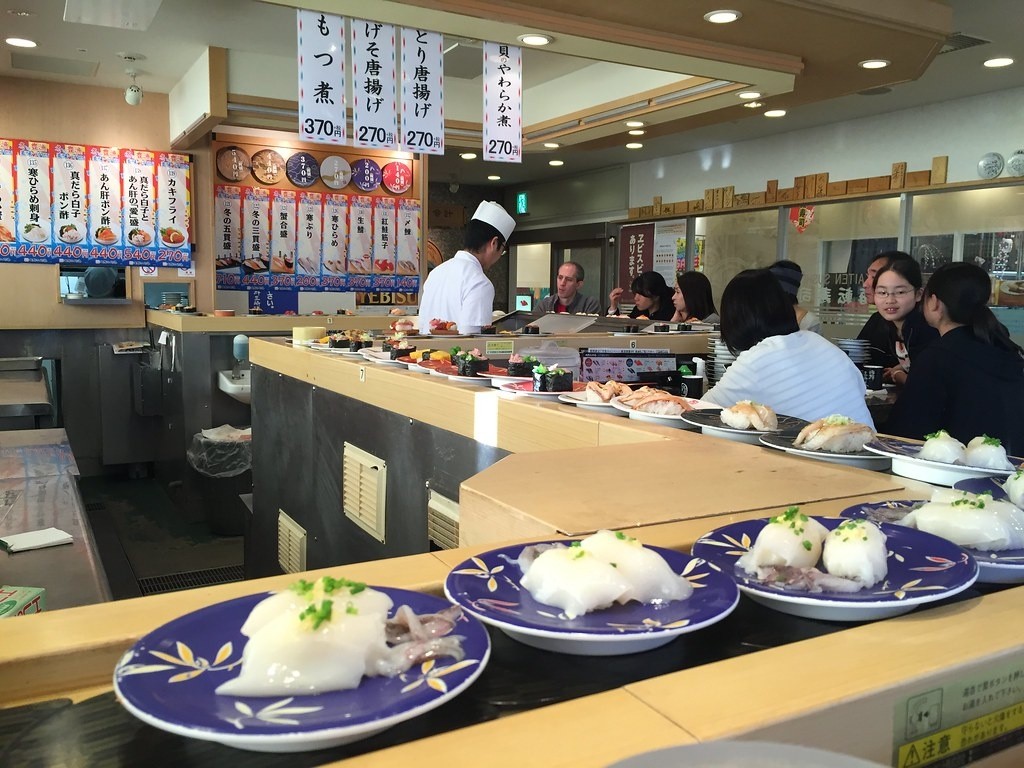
[
  {"x1": 875, "y1": 287, "x2": 915, "y2": 298},
  {"x1": 497, "y1": 240, "x2": 507, "y2": 256}
]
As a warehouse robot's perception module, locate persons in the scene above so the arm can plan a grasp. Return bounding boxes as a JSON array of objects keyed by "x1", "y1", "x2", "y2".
[
  {"x1": 881, "y1": 261, "x2": 1024, "y2": 459},
  {"x1": 671, "y1": 271, "x2": 721, "y2": 324},
  {"x1": 532, "y1": 260, "x2": 602, "y2": 317},
  {"x1": 766, "y1": 260, "x2": 823, "y2": 339},
  {"x1": 854, "y1": 251, "x2": 941, "y2": 392},
  {"x1": 418, "y1": 199, "x2": 518, "y2": 334},
  {"x1": 606, "y1": 270, "x2": 676, "y2": 322},
  {"x1": 699, "y1": 268, "x2": 878, "y2": 435}
]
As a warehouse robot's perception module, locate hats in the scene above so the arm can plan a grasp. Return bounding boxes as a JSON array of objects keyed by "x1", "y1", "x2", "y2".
[
  {"x1": 85, "y1": 266, "x2": 116, "y2": 297},
  {"x1": 470, "y1": 200, "x2": 516, "y2": 242},
  {"x1": 767, "y1": 260, "x2": 803, "y2": 286}
]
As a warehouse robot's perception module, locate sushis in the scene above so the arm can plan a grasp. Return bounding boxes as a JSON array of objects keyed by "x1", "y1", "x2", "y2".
[
  {"x1": 213, "y1": 509, "x2": 886, "y2": 695},
  {"x1": 144, "y1": 303, "x2": 196, "y2": 312},
  {"x1": 317, "y1": 318, "x2": 574, "y2": 393},
  {"x1": 898, "y1": 430, "x2": 1023, "y2": 554},
  {"x1": 249, "y1": 308, "x2": 407, "y2": 315},
  {"x1": 585, "y1": 379, "x2": 694, "y2": 415},
  {"x1": 606, "y1": 314, "x2": 721, "y2": 333},
  {"x1": 719, "y1": 399, "x2": 878, "y2": 454},
  {"x1": 217, "y1": 256, "x2": 416, "y2": 274}
]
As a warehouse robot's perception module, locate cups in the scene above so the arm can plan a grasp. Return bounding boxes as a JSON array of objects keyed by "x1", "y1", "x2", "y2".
[
  {"x1": 681, "y1": 375, "x2": 704, "y2": 400},
  {"x1": 864, "y1": 366, "x2": 883, "y2": 390}
]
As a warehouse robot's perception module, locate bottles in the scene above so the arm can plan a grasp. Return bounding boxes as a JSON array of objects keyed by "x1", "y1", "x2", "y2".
[{"x1": 692, "y1": 357, "x2": 709, "y2": 394}]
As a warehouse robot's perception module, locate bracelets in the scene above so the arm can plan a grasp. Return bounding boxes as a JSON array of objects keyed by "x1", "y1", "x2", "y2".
[{"x1": 891, "y1": 369, "x2": 907, "y2": 384}]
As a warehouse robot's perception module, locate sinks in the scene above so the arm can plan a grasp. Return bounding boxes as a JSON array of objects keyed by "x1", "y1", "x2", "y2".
[{"x1": 217, "y1": 369, "x2": 251, "y2": 404}]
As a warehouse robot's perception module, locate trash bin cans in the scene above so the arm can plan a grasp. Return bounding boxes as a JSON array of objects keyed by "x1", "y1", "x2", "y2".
[{"x1": 193, "y1": 426, "x2": 252, "y2": 535}]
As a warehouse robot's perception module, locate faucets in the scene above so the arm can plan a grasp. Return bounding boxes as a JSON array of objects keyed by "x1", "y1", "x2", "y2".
[{"x1": 232, "y1": 358, "x2": 245, "y2": 380}]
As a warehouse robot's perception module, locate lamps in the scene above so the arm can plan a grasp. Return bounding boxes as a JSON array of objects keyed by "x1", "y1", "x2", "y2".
[{"x1": 123, "y1": 66, "x2": 145, "y2": 106}]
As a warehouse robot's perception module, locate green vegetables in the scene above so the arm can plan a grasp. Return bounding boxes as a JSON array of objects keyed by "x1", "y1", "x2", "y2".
[{"x1": 25, "y1": 224, "x2": 172, "y2": 236}]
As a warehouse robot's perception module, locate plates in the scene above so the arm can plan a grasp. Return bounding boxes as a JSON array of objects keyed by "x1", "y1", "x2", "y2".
[
  {"x1": 22, "y1": 236, "x2": 186, "y2": 248},
  {"x1": 245, "y1": 313, "x2": 358, "y2": 316},
  {"x1": 1000, "y1": 280, "x2": 1024, "y2": 295},
  {"x1": 679, "y1": 409, "x2": 812, "y2": 445},
  {"x1": 284, "y1": 336, "x2": 383, "y2": 354},
  {"x1": 500, "y1": 382, "x2": 568, "y2": 403},
  {"x1": 952, "y1": 476, "x2": 1024, "y2": 511},
  {"x1": 977, "y1": 148, "x2": 1024, "y2": 179},
  {"x1": 620, "y1": 304, "x2": 637, "y2": 313},
  {"x1": 217, "y1": 145, "x2": 412, "y2": 194},
  {"x1": 613, "y1": 330, "x2": 712, "y2": 336},
  {"x1": 444, "y1": 539, "x2": 740, "y2": 656},
  {"x1": 832, "y1": 338, "x2": 872, "y2": 361},
  {"x1": 375, "y1": 333, "x2": 552, "y2": 337},
  {"x1": 610, "y1": 395, "x2": 724, "y2": 428},
  {"x1": 156, "y1": 292, "x2": 202, "y2": 316},
  {"x1": 358, "y1": 347, "x2": 491, "y2": 386},
  {"x1": 113, "y1": 585, "x2": 492, "y2": 752},
  {"x1": 758, "y1": 430, "x2": 909, "y2": 471},
  {"x1": 706, "y1": 337, "x2": 738, "y2": 388},
  {"x1": 690, "y1": 516, "x2": 980, "y2": 621},
  {"x1": 839, "y1": 500, "x2": 1024, "y2": 584},
  {"x1": 862, "y1": 441, "x2": 1024, "y2": 486},
  {"x1": 557, "y1": 395, "x2": 630, "y2": 416},
  {"x1": 477, "y1": 372, "x2": 533, "y2": 387}
]
